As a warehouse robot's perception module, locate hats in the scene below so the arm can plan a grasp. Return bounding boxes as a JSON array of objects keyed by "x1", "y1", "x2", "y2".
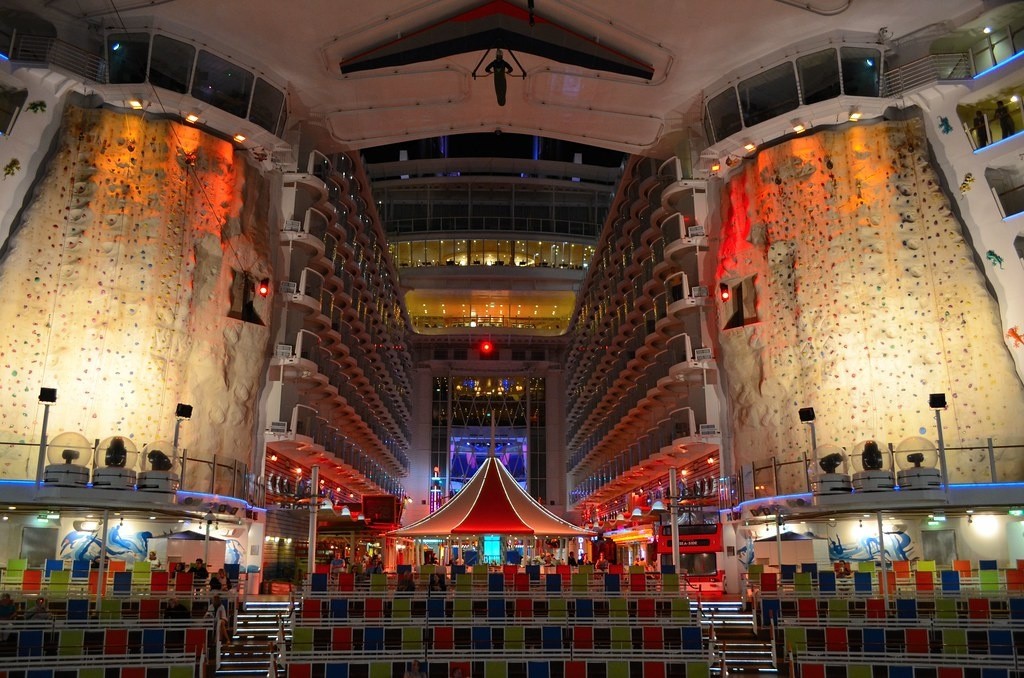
[{"x1": 150, "y1": 549, "x2": 157, "y2": 555}]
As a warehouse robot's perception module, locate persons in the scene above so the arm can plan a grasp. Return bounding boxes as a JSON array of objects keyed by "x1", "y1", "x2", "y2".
[
  {"x1": 835, "y1": 559, "x2": 852, "y2": 599},
  {"x1": 0, "y1": 593, "x2": 52, "y2": 645},
  {"x1": 429, "y1": 574, "x2": 446, "y2": 600},
  {"x1": 171, "y1": 559, "x2": 232, "y2": 599},
  {"x1": 164, "y1": 598, "x2": 191, "y2": 644},
  {"x1": 453, "y1": 667, "x2": 469, "y2": 678},
  {"x1": 93, "y1": 549, "x2": 111, "y2": 569},
  {"x1": 404, "y1": 660, "x2": 426, "y2": 678},
  {"x1": 204, "y1": 596, "x2": 230, "y2": 645},
  {"x1": 521, "y1": 552, "x2": 609, "y2": 572},
  {"x1": 330, "y1": 552, "x2": 415, "y2": 599},
  {"x1": 143, "y1": 549, "x2": 161, "y2": 570}
]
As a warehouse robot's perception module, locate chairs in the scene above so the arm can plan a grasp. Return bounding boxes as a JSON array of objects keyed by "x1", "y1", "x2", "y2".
[
  {"x1": 747, "y1": 558, "x2": 1024, "y2": 678},
  {"x1": 0, "y1": 558, "x2": 261, "y2": 678},
  {"x1": 598, "y1": 477, "x2": 713, "y2": 522},
  {"x1": 286, "y1": 564, "x2": 714, "y2": 678},
  {"x1": 271, "y1": 478, "x2": 336, "y2": 508}
]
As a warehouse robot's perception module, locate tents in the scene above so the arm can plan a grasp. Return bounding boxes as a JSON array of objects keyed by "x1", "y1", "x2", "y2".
[{"x1": 147, "y1": 531, "x2": 226, "y2": 571}]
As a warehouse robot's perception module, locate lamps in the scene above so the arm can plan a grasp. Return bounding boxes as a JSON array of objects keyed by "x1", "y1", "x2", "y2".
[
  {"x1": 688, "y1": 225, "x2": 716, "y2": 437},
  {"x1": 712, "y1": 111, "x2": 862, "y2": 171},
  {"x1": 798, "y1": 407, "x2": 816, "y2": 424},
  {"x1": 929, "y1": 393, "x2": 948, "y2": 410},
  {"x1": 270, "y1": 219, "x2": 301, "y2": 434},
  {"x1": 174, "y1": 404, "x2": 192, "y2": 422},
  {"x1": 37, "y1": 386, "x2": 57, "y2": 406}
]
